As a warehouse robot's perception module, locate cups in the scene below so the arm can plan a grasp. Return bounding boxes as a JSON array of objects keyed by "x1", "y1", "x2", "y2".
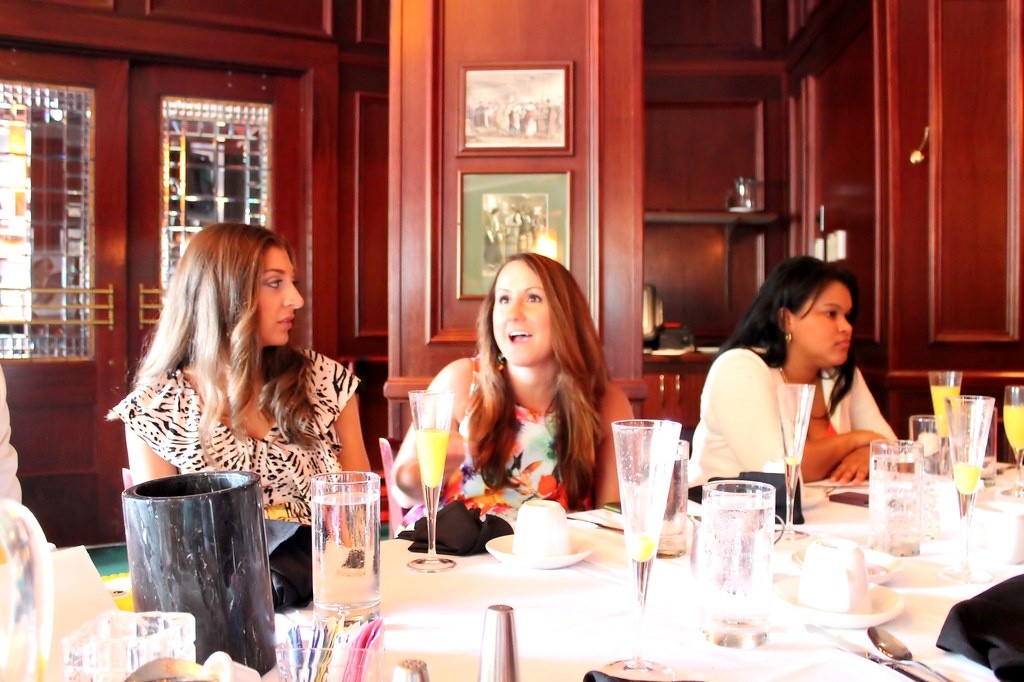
[
  {"x1": 655, "y1": 440, "x2": 687, "y2": 559},
  {"x1": 795, "y1": 538, "x2": 875, "y2": 616},
  {"x1": 310, "y1": 472, "x2": 383, "y2": 626},
  {"x1": 864, "y1": 439, "x2": 925, "y2": 558},
  {"x1": 690, "y1": 478, "x2": 777, "y2": 648},
  {"x1": 513, "y1": 500, "x2": 570, "y2": 562},
  {"x1": 60, "y1": 608, "x2": 197, "y2": 682},
  {"x1": 908, "y1": 414, "x2": 952, "y2": 502},
  {"x1": 724, "y1": 174, "x2": 756, "y2": 212},
  {"x1": 966, "y1": 405, "x2": 998, "y2": 488}
]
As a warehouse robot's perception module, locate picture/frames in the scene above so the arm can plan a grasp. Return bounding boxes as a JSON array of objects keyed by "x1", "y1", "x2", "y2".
[
  {"x1": 460, "y1": 60, "x2": 576, "y2": 161},
  {"x1": 453, "y1": 169, "x2": 571, "y2": 301}
]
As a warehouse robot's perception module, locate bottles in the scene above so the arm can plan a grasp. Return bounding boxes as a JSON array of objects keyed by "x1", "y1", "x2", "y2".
[{"x1": 121, "y1": 469, "x2": 278, "y2": 677}]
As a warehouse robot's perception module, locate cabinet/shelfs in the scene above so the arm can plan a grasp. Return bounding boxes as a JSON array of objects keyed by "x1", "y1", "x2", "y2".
[{"x1": 642, "y1": 209, "x2": 789, "y2": 361}]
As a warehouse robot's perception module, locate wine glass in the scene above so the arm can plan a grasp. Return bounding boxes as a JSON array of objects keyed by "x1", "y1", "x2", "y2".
[
  {"x1": 407, "y1": 389, "x2": 456, "y2": 573},
  {"x1": 922, "y1": 373, "x2": 1023, "y2": 574},
  {"x1": 772, "y1": 382, "x2": 815, "y2": 545},
  {"x1": 602, "y1": 420, "x2": 683, "y2": 681}
]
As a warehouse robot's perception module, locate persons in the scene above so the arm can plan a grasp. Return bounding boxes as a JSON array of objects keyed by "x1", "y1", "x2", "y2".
[
  {"x1": 689, "y1": 256, "x2": 898, "y2": 492},
  {"x1": 107, "y1": 223, "x2": 371, "y2": 523},
  {"x1": 393, "y1": 252, "x2": 635, "y2": 524}
]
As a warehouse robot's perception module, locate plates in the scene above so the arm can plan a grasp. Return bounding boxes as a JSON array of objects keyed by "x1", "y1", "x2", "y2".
[
  {"x1": 775, "y1": 574, "x2": 904, "y2": 631},
  {"x1": 487, "y1": 533, "x2": 594, "y2": 568}
]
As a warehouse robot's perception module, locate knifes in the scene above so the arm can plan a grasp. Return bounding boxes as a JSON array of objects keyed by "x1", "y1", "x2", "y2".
[{"x1": 803, "y1": 622, "x2": 946, "y2": 682}]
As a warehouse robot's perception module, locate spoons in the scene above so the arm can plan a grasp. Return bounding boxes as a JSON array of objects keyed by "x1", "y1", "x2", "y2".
[{"x1": 866, "y1": 625, "x2": 957, "y2": 682}]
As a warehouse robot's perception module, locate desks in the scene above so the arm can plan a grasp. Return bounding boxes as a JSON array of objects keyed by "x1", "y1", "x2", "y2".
[{"x1": 265, "y1": 476, "x2": 1024, "y2": 682}]
